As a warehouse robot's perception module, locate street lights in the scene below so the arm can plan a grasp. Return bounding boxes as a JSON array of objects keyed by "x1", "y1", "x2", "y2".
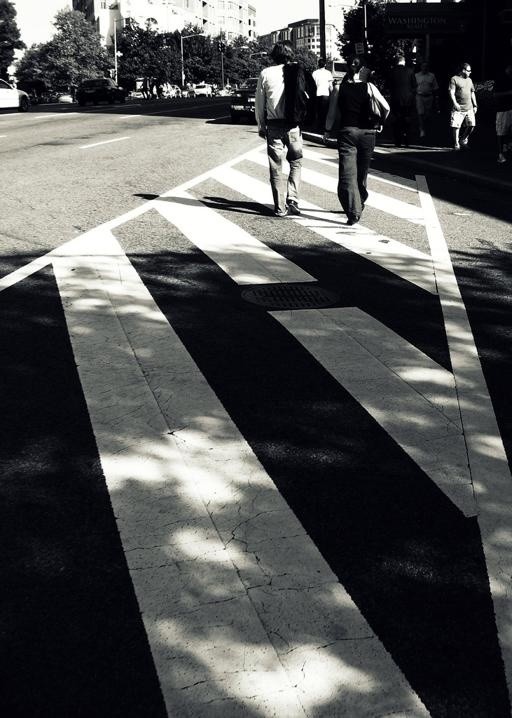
[
  {"x1": 180, "y1": 32, "x2": 208, "y2": 87},
  {"x1": 327, "y1": 2, "x2": 368, "y2": 39},
  {"x1": 219, "y1": 45, "x2": 250, "y2": 89},
  {"x1": 248, "y1": 52, "x2": 267, "y2": 78},
  {"x1": 310, "y1": 22, "x2": 342, "y2": 37},
  {"x1": 113, "y1": 14, "x2": 140, "y2": 83}
]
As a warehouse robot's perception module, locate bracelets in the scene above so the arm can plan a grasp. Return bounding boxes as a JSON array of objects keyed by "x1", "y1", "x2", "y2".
[{"x1": 324, "y1": 129, "x2": 331, "y2": 134}]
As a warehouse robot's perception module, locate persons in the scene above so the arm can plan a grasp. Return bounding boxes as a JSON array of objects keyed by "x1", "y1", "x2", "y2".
[
  {"x1": 383, "y1": 58, "x2": 512, "y2": 164},
  {"x1": 310, "y1": 59, "x2": 334, "y2": 128},
  {"x1": 254, "y1": 40, "x2": 317, "y2": 216},
  {"x1": 323, "y1": 57, "x2": 390, "y2": 224},
  {"x1": 140, "y1": 78, "x2": 220, "y2": 101}
]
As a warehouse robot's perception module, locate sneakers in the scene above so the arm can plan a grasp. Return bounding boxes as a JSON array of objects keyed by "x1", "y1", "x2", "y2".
[
  {"x1": 347, "y1": 216, "x2": 358, "y2": 225},
  {"x1": 288, "y1": 200, "x2": 300, "y2": 214}
]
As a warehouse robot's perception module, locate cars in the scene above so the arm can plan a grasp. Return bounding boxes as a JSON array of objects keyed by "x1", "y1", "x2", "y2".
[
  {"x1": 229, "y1": 77, "x2": 260, "y2": 123},
  {"x1": 0, "y1": 78, "x2": 30, "y2": 113},
  {"x1": 127, "y1": 78, "x2": 239, "y2": 103},
  {"x1": 77, "y1": 79, "x2": 127, "y2": 105},
  {"x1": 15, "y1": 80, "x2": 59, "y2": 105}
]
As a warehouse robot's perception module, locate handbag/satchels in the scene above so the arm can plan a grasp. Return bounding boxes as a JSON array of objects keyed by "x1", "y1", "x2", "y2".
[
  {"x1": 369, "y1": 98, "x2": 384, "y2": 129},
  {"x1": 282, "y1": 63, "x2": 308, "y2": 124}
]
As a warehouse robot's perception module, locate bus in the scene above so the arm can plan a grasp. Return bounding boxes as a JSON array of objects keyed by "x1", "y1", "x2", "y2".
[{"x1": 331, "y1": 60, "x2": 347, "y2": 82}]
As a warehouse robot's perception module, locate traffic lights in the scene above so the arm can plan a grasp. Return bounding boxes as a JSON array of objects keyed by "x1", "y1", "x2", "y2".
[
  {"x1": 217, "y1": 40, "x2": 221, "y2": 52},
  {"x1": 222, "y1": 40, "x2": 226, "y2": 52}
]
